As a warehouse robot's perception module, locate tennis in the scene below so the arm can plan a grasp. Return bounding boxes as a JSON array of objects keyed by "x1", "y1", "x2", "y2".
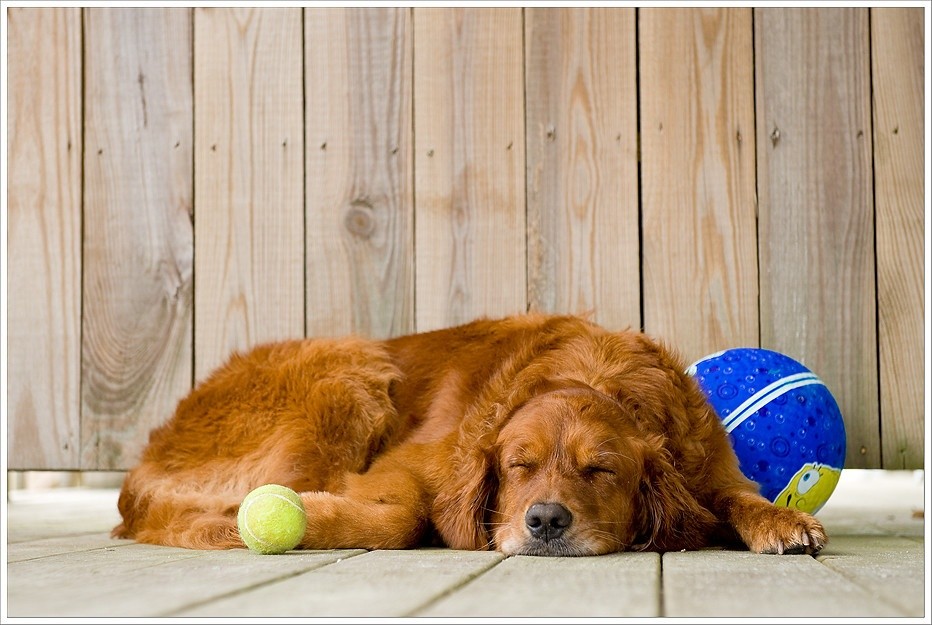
[{"x1": 236, "y1": 484, "x2": 306, "y2": 556}]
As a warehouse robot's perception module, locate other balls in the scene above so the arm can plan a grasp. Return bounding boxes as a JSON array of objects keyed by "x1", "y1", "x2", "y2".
[{"x1": 681, "y1": 347, "x2": 848, "y2": 519}]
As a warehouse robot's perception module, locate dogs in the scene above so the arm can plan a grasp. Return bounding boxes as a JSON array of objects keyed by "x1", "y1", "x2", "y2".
[{"x1": 111, "y1": 311, "x2": 829, "y2": 557}]
